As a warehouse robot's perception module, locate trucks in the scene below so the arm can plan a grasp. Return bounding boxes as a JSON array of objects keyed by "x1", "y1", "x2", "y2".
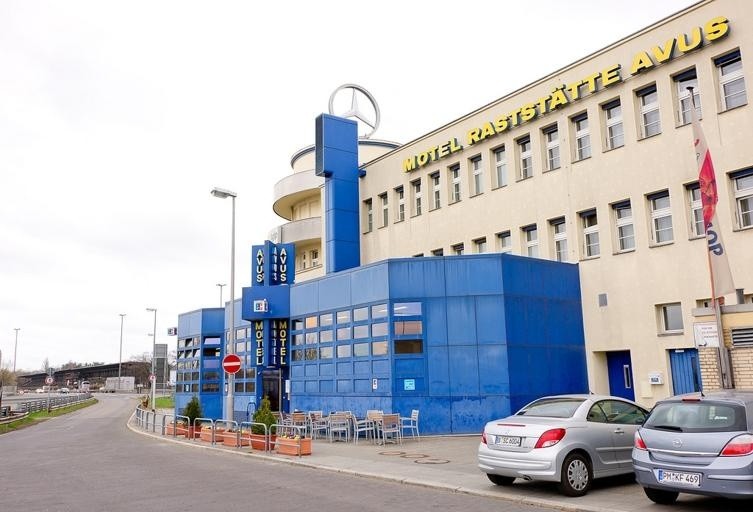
[{"x1": 99, "y1": 376, "x2": 135, "y2": 394}]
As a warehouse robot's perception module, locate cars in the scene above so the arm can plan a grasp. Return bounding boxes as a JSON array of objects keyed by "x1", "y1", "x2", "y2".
[
  {"x1": 630, "y1": 388, "x2": 752, "y2": 506},
  {"x1": 15, "y1": 386, "x2": 72, "y2": 395},
  {"x1": 475, "y1": 393, "x2": 652, "y2": 497}
]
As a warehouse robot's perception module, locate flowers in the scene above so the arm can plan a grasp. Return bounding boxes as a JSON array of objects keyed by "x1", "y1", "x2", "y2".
[
  {"x1": 223, "y1": 428, "x2": 247, "y2": 433},
  {"x1": 170, "y1": 419, "x2": 184, "y2": 425},
  {"x1": 202, "y1": 426, "x2": 221, "y2": 430}
]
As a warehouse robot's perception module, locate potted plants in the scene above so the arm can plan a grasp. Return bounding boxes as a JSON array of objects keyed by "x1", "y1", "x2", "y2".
[
  {"x1": 249, "y1": 395, "x2": 278, "y2": 451},
  {"x1": 181, "y1": 395, "x2": 203, "y2": 439}
]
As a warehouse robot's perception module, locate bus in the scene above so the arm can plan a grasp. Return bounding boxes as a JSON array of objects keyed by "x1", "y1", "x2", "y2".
[{"x1": 80, "y1": 381, "x2": 91, "y2": 392}]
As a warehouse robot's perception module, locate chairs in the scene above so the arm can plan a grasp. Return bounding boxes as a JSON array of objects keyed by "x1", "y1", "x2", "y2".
[{"x1": 272, "y1": 410, "x2": 420, "y2": 449}]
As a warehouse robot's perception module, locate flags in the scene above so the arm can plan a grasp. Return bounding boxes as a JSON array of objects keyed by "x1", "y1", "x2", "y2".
[{"x1": 689, "y1": 94, "x2": 735, "y2": 301}]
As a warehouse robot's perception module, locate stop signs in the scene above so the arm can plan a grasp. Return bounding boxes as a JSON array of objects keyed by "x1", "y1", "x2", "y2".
[{"x1": 221, "y1": 354, "x2": 241, "y2": 375}]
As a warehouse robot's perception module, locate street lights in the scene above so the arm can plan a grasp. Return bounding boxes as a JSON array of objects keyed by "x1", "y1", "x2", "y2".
[
  {"x1": 12, "y1": 326, "x2": 21, "y2": 386},
  {"x1": 214, "y1": 282, "x2": 226, "y2": 311},
  {"x1": 117, "y1": 311, "x2": 125, "y2": 391},
  {"x1": 146, "y1": 306, "x2": 157, "y2": 396},
  {"x1": 206, "y1": 184, "x2": 238, "y2": 431}
]
